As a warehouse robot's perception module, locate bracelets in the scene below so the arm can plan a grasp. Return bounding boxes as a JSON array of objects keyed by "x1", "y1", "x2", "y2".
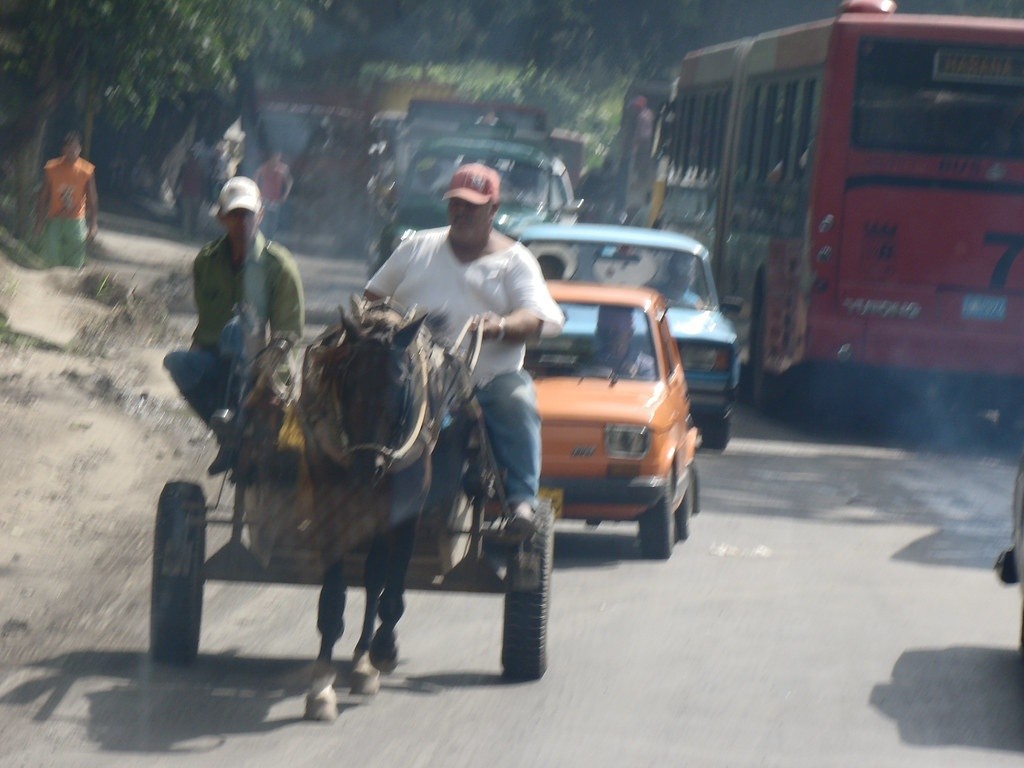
[{"x1": 494, "y1": 312, "x2": 508, "y2": 344}]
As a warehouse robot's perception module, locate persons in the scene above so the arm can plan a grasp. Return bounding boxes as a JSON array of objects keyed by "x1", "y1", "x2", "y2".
[
  {"x1": 641, "y1": 254, "x2": 710, "y2": 312},
  {"x1": 765, "y1": 157, "x2": 786, "y2": 183},
  {"x1": 171, "y1": 149, "x2": 208, "y2": 242},
  {"x1": 625, "y1": 91, "x2": 659, "y2": 175},
  {"x1": 583, "y1": 304, "x2": 660, "y2": 385},
  {"x1": 211, "y1": 137, "x2": 243, "y2": 206},
  {"x1": 362, "y1": 163, "x2": 568, "y2": 545},
  {"x1": 33, "y1": 130, "x2": 101, "y2": 269},
  {"x1": 248, "y1": 150, "x2": 298, "y2": 243},
  {"x1": 796, "y1": 147, "x2": 811, "y2": 173},
  {"x1": 161, "y1": 169, "x2": 304, "y2": 478}
]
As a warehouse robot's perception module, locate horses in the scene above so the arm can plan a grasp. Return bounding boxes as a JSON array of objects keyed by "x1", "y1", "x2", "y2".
[{"x1": 293, "y1": 292, "x2": 455, "y2": 722}]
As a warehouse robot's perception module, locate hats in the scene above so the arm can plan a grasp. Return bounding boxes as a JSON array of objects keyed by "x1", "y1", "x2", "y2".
[
  {"x1": 443, "y1": 163, "x2": 500, "y2": 205},
  {"x1": 219, "y1": 176, "x2": 260, "y2": 217}
]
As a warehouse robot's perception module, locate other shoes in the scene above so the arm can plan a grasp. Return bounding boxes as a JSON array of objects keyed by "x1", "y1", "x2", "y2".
[
  {"x1": 506, "y1": 504, "x2": 535, "y2": 531},
  {"x1": 207, "y1": 426, "x2": 243, "y2": 478}
]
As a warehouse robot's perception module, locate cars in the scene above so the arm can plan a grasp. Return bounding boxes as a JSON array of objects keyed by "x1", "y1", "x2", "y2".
[
  {"x1": 470, "y1": 279, "x2": 703, "y2": 562},
  {"x1": 504, "y1": 220, "x2": 734, "y2": 457},
  {"x1": 160, "y1": 86, "x2": 608, "y2": 293}
]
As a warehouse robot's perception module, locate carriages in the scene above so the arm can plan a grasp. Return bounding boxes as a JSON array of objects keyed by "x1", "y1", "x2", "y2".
[{"x1": 147, "y1": 282, "x2": 563, "y2": 719}]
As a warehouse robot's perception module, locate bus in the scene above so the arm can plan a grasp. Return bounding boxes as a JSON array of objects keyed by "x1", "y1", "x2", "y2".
[{"x1": 636, "y1": 5, "x2": 1024, "y2": 451}]
